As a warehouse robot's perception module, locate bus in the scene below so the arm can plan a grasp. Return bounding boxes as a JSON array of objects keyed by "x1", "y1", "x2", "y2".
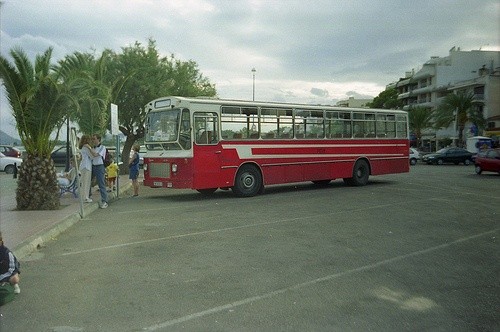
[{"x1": 137, "y1": 95, "x2": 410, "y2": 197}]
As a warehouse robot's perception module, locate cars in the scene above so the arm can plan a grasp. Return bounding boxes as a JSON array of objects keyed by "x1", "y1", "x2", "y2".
[
  {"x1": 409, "y1": 148, "x2": 420, "y2": 165},
  {"x1": 476, "y1": 148, "x2": 500, "y2": 176},
  {"x1": 422, "y1": 146, "x2": 473, "y2": 166},
  {"x1": 50, "y1": 145, "x2": 74, "y2": 167},
  {"x1": 104, "y1": 150, "x2": 124, "y2": 166},
  {"x1": 0, "y1": 145, "x2": 24, "y2": 174},
  {"x1": 130, "y1": 146, "x2": 147, "y2": 165}
]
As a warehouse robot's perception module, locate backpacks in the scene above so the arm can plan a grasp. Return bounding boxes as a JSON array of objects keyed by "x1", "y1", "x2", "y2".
[{"x1": 101, "y1": 149, "x2": 112, "y2": 167}]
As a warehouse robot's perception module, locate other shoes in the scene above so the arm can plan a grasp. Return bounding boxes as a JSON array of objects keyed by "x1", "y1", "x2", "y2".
[
  {"x1": 13, "y1": 282, "x2": 21, "y2": 293},
  {"x1": 83, "y1": 198, "x2": 92, "y2": 203},
  {"x1": 106, "y1": 189, "x2": 113, "y2": 192},
  {"x1": 100, "y1": 201, "x2": 108, "y2": 208},
  {"x1": 112, "y1": 187, "x2": 116, "y2": 191},
  {"x1": 130, "y1": 194, "x2": 139, "y2": 198}
]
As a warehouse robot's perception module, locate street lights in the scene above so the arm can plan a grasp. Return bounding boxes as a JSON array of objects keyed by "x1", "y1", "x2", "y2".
[{"x1": 251, "y1": 68, "x2": 257, "y2": 102}]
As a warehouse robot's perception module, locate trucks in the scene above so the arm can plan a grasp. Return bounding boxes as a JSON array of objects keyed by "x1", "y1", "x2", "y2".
[{"x1": 466, "y1": 136, "x2": 493, "y2": 161}]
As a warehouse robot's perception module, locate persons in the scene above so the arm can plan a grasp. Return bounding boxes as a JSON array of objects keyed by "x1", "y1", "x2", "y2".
[
  {"x1": 0, "y1": 233, "x2": 21, "y2": 293},
  {"x1": 128, "y1": 144, "x2": 140, "y2": 198},
  {"x1": 105, "y1": 158, "x2": 119, "y2": 192},
  {"x1": 79, "y1": 134, "x2": 93, "y2": 202},
  {"x1": 97, "y1": 150, "x2": 113, "y2": 190},
  {"x1": 84, "y1": 134, "x2": 108, "y2": 208}
]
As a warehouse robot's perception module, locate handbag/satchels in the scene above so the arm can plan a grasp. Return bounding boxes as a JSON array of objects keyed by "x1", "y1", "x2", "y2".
[{"x1": 0, "y1": 281, "x2": 15, "y2": 305}]
